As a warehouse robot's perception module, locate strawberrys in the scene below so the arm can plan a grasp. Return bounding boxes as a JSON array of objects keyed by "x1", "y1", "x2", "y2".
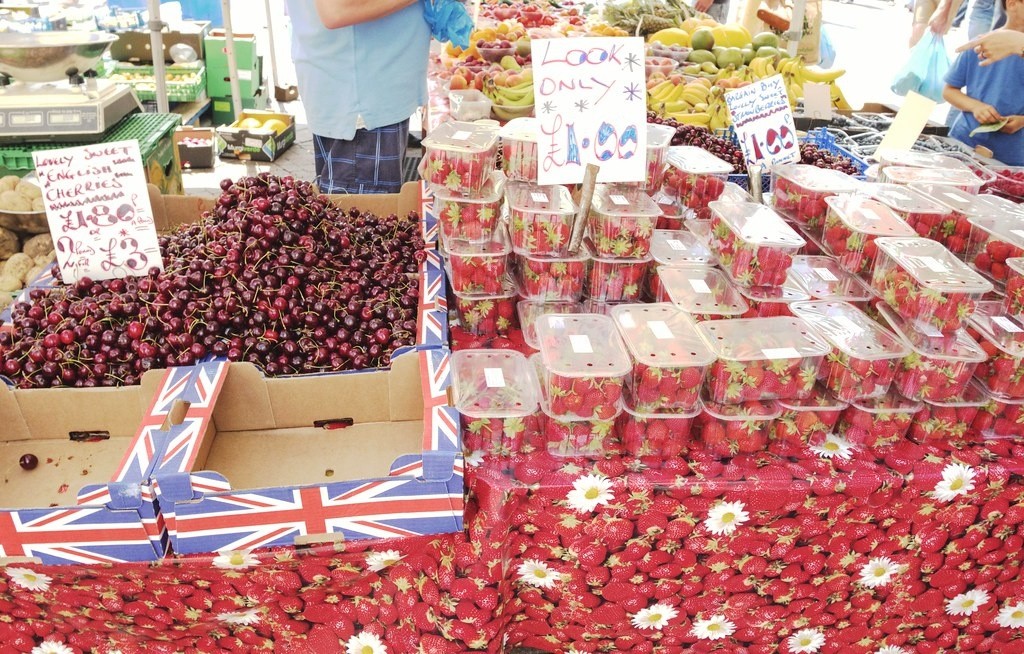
[
  {"x1": 0, "y1": 455, "x2": 1024, "y2": 654},
  {"x1": 423, "y1": 133, "x2": 1024, "y2": 456}
]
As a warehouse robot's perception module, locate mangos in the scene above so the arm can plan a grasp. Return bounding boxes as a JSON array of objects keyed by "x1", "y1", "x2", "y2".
[{"x1": 688, "y1": 30, "x2": 790, "y2": 69}]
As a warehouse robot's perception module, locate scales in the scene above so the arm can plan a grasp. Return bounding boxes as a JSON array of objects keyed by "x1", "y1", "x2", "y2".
[{"x1": 0, "y1": 31, "x2": 141, "y2": 144}]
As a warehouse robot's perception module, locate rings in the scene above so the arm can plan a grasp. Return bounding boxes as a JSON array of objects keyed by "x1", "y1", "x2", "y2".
[
  {"x1": 981, "y1": 51, "x2": 986, "y2": 60},
  {"x1": 979, "y1": 43, "x2": 984, "y2": 51}
]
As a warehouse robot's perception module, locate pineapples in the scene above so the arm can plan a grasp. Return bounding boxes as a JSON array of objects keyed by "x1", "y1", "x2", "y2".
[{"x1": 603, "y1": 0, "x2": 694, "y2": 33}]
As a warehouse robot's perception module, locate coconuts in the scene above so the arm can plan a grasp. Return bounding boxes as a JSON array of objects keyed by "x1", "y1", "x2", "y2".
[{"x1": 649, "y1": 18, "x2": 751, "y2": 49}]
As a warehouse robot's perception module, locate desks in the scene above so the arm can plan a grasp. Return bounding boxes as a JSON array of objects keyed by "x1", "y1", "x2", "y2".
[{"x1": 1, "y1": 439, "x2": 1024, "y2": 654}]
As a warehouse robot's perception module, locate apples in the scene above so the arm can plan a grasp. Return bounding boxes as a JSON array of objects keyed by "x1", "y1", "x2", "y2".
[{"x1": 439, "y1": 39, "x2": 532, "y2": 90}]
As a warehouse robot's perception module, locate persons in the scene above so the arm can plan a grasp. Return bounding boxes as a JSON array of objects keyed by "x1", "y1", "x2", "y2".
[
  {"x1": 690, "y1": 0, "x2": 730, "y2": 26},
  {"x1": 283, "y1": 0, "x2": 432, "y2": 195},
  {"x1": 953, "y1": 29, "x2": 1024, "y2": 67},
  {"x1": 906, "y1": 0, "x2": 1007, "y2": 110},
  {"x1": 941, "y1": 0, "x2": 1024, "y2": 167}
]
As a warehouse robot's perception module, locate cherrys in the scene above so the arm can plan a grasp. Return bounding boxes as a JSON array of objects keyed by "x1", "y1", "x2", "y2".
[
  {"x1": 647, "y1": 109, "x2": 857, "y2": 189},
  {"x1": 0, "y1": 175, "x2": 427, "y2": 386}
]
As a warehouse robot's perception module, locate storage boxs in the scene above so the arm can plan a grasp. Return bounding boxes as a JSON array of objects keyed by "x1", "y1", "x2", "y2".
[
  {"x1": 111, "y1": 20, "x2": 211, "y2": 60},
  {"x1": 0, "y1": 113, "x2": 184, "y2": 195},
  {"x1": 174, "y1": 126, "x2": 215, "y2": 166},
  {"x1": 108, "y1": 66, "x2": 205, "y2": 102},
  {"x1": 449, "y1": 88, "x2": 492, "y2": 122},
  {"x1": 0, "y1": 183, "x2": 465, "y2": 564},
  {"x1": 216, "y1": 108, "x2": 296, "y2": 162},
  {"x1": 422, "y1": 104, "x2": 1023, "y2": 456},
  {"x1": 204, "y1": 29, "x2": 268, "y2": 124}
]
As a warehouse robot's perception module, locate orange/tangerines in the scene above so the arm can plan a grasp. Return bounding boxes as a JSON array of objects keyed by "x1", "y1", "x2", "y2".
[{"x1": 446, "y1": 25, "x2": 521, "y2": 61}]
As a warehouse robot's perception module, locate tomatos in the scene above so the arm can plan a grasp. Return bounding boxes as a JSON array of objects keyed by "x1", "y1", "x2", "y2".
[{"x1": 483, "y1": 4, "x2": 583, "y2": 29}]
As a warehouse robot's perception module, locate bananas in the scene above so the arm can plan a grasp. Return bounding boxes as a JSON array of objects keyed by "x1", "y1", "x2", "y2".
[
  {"x1": 646, "y1": 55, "x2": 846, "y2": 131},
  {"x1": 480, "y1": 76, "x2": 534, "y2": 119}
]
